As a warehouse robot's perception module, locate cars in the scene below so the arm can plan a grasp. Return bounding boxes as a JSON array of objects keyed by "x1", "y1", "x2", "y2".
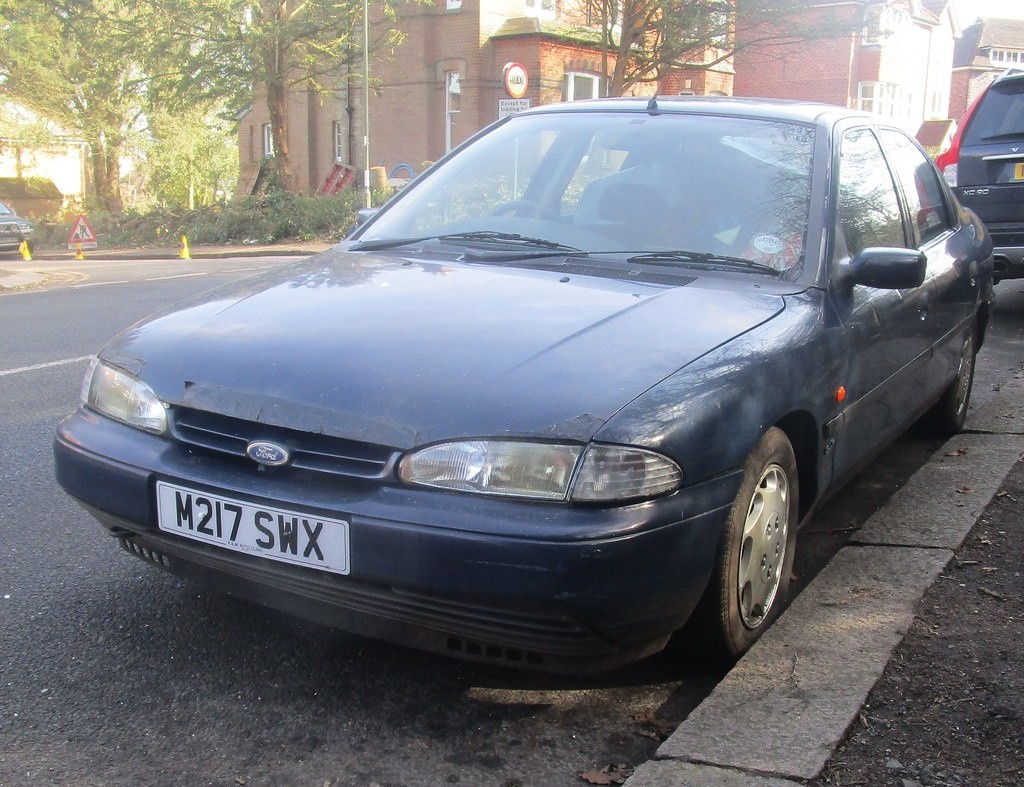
[
  {"x1": 0, "y1": 199, "x2": 34, "y2": 252},
  {"x1": 46, "y1": 93, "x2": 998, "y2": 674}
]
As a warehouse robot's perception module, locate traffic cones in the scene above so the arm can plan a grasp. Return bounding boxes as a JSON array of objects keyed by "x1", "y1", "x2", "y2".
[
  {"x1": 179, "y1": 235, "x2": 193, "y2": 259},
  {"x1": 20, "y1": 239, "x2": 32, "y2": 261},
  {"x1": 74, "y1": 240, "x2": 85, "y2": 260}
]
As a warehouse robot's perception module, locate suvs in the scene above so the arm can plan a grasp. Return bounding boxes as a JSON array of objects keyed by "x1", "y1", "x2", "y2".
[{"x1": 936, "y1": 68, "x2": 1024, "y2": 285}]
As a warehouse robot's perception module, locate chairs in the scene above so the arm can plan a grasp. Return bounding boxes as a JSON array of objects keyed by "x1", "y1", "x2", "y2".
[{"x1": 586, "y1": 182, "x2": 660, "y2": 250}]
